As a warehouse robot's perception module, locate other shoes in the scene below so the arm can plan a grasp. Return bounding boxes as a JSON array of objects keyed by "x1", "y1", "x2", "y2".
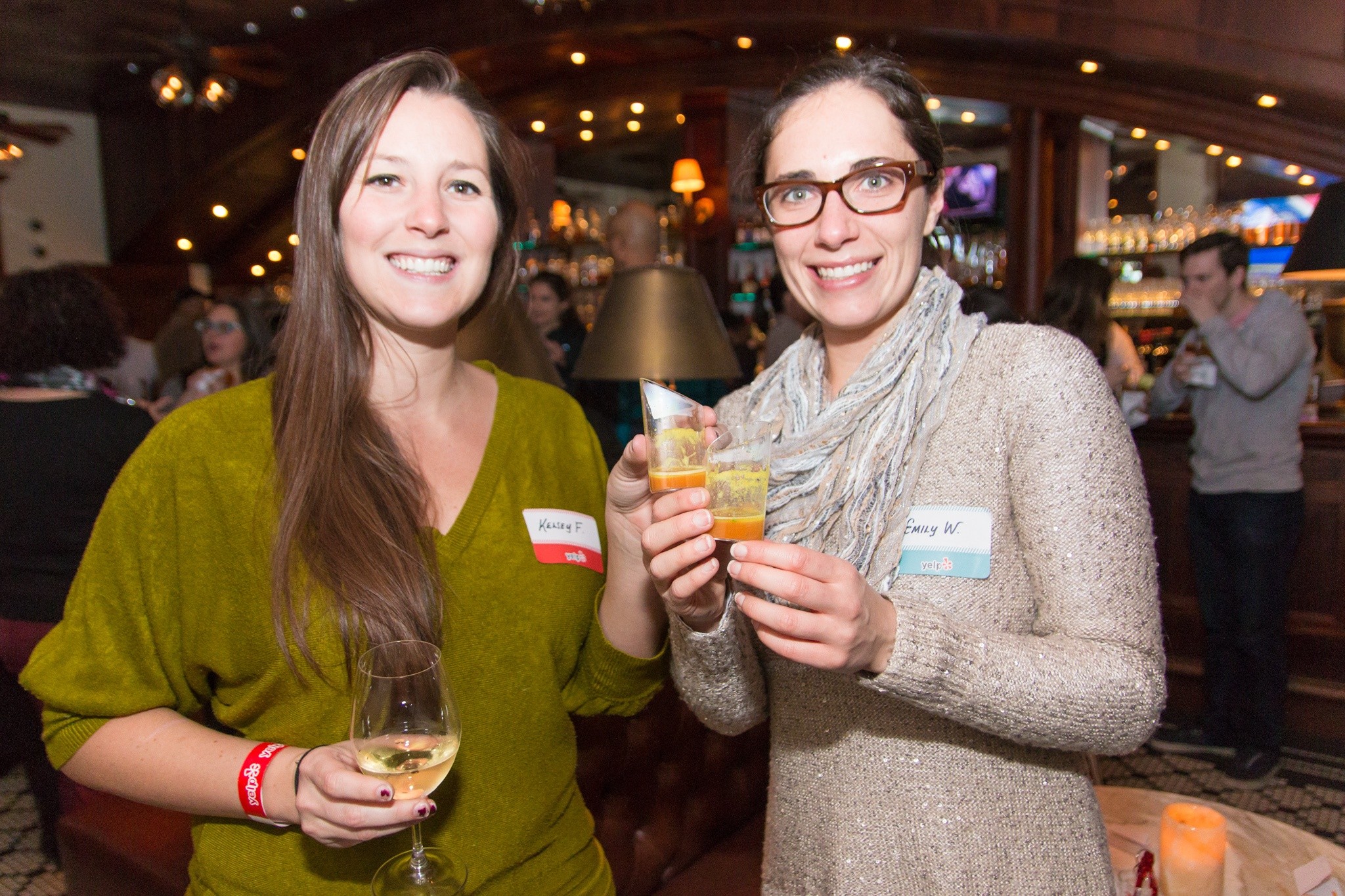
[
  {"x1": 1147, "y1": 711, "x2": 1241, "y2": 757},
  {"x1": 1218, "y1": 744, "x2": 1285, "y2": 789}
]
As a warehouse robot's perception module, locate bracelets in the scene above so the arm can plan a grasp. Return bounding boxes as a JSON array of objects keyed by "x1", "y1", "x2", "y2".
[
  {"x1": 295, "y1": 744, "x2": 329, "y2": 795},
  {"x1": 238, "y1": 742, "x2": 294, "y2": 828}
]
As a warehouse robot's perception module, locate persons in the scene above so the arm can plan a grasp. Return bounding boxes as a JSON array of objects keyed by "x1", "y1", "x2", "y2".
[
  {"x1": 19, "y1": 52, "x2": 731, "y2": 893},
  {"x1": 644, "y1": 50, "x2": 1168, "y2": 896},
  {"x1": 1147, "y1": 231, "x2": 1317, "y2": 782},
  {"x1": 1, "y1": 263, "x2": 816, "y2": 623},
  {"x1": 1020, "y1": 253, "x2": 1144, "y2": 413}
]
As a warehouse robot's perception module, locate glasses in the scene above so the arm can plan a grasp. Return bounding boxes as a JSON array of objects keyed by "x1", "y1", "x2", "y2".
[
  {"x1": 198, "y1": 316, "x2": 245, "y2": 333},
  {"x1": 750, "y1": 161, "x2": 934, "y2": 228}
]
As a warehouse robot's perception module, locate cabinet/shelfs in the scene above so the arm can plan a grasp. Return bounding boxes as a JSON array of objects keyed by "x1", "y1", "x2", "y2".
[{"x1": 1080, "y1": 242, "x2": 1344, "y2": 329}]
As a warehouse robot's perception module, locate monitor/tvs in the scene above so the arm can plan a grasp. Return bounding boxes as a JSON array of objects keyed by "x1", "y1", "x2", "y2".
[{"x1": 934, "y1": 165, "x2": 998, "y2": 225}]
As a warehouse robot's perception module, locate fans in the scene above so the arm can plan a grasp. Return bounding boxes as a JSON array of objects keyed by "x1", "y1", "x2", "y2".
[
  {"x1": 67, "y1": 0, "x2": 288, "y2": 85},
  {"x1": 0, "y1": 110, "x2": 72, "y2": 160}
]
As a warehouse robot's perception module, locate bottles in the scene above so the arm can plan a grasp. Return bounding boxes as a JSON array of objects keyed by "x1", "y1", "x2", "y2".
[
  {"x1": 925, "y1": 229, "x2": 1008, "y2": 289},
  {"x1": 520, "y1": 198, "x2": 786, "y2": 349},
  {"x1": 1081, "y1": 201, "x2": 1326, "y2": 373}
]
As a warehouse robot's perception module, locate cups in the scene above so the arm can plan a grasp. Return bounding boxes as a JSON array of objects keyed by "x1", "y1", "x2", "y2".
[
  {"x1": 639, "y1": 376, "x2": 709, "y2": 492},
  {"x1": 710, "y1": 436, "x2": 772, "y2": 541}
]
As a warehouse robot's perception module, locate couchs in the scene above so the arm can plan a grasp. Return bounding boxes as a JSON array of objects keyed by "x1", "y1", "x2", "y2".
[{"x1": 59, "y1": 682, "x2": 769, "y2": 896}]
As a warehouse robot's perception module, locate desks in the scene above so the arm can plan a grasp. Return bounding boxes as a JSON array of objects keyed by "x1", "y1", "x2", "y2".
[{"x1": 1091, "y1": 786, "x2": 1345, "y2": 896}]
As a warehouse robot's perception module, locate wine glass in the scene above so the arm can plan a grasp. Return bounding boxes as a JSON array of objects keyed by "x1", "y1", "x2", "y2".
[{"x1": 348, "y1": 639, "x2": 468, "y2": 896}]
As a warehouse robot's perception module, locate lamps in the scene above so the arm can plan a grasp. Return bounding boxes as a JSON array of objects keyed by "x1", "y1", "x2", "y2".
[
  {"x1": 571, "y1": 267, "x2": 743, "y2": 381},
  {"x1": 672, "y1": 158, "x2": 706, "y2": 208},
  {"x1": 153, "y1": 68, "x2": 237, "y2": 111},
  {"x1": 0, "y1": 138, "x2": 23, "y2": 161},
  {"x1": 1278, "y1": 182, "x2": 1345, "y2": 282}
]
